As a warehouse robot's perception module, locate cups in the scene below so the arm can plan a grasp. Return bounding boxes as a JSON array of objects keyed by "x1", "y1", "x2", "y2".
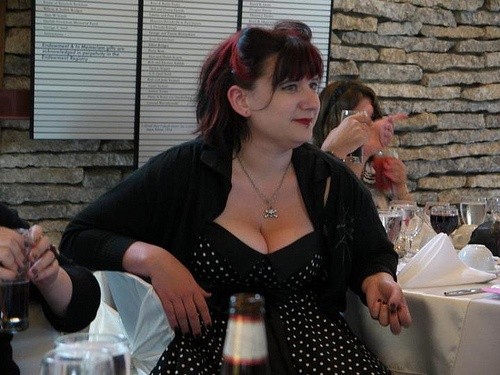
[
  {"x1": 377, "y1": 209, "x2": 402, "y2": 247},
  {"x1": 424, "y1": 202, "x2": 450, "y2": 236},
  {"x1": 53, "y1": 332, "x2": 135, "y2": 375},
  {"x1": 0, "y1": 228, "x2": 31, "y2": 333},
  {"x1": 429, "y1": 207, "x2": 458, "y2": 237},
  {"x1": 340, "y1": 110, "x2": 367, "y2": 164},
  {"x1": 478, "y1": 196, "x2": 500, "y2": 222},
  {"x1": 460, "y1": 195, "x2": 487, "y2": 228},
  {"x1": 38, "y1": 347, "x2": 115, "y2": 375}
]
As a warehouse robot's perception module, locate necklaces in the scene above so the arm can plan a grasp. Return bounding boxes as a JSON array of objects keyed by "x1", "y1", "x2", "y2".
[{"x1": 235, "y1": 152, "x2": 290, "y2": 220}]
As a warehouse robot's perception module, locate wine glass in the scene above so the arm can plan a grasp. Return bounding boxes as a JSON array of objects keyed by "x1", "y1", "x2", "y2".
[
  {"x1": 389, "y1": 200, "x2": 417, "y2": 258},
  {"x1": 391, "y1": 205, "x2": 426, "y2": 263}
]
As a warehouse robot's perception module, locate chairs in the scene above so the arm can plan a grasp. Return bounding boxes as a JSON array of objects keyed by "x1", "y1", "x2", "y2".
[{"x1": 103, "y1": 271, "x2": 175, "y2": 375}]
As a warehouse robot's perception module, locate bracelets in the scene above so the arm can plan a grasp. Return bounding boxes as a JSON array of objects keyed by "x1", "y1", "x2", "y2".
[{"x1": 325, "y1": 151, "x2": 345, "y2": 162}]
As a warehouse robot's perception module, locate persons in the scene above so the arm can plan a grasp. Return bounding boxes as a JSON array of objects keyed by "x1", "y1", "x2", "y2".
[
  {"x1": 312, "y1": 80, "x2": 411, "y2": 259},
  {"x1": 59, "y1": 20, "x2": 411, "y2": 375},
  {"x1": 0, "y1": 206, "x2": 101, "y2": 375}
]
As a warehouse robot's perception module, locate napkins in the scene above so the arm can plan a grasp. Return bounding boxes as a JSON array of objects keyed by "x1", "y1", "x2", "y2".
[{"x1": 398, "y1": 232, "x2": 496, "y2": 290}]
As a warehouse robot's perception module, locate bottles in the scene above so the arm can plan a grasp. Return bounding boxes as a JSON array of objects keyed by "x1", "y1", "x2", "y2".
[{"x1": 220, "y1": 291, "x2": 271, "y2": 375}]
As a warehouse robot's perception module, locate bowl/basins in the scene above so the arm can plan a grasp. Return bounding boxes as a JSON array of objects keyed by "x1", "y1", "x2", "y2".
[
  {"x1": 448, "y1": 223, "x2": 477, "y2": 250},
  {"x1": 467, "y1": 221, "x2": 500, "y2": 257},
  {"x1": 458, "y1": 244, "x2": 498, "y2": 273}
]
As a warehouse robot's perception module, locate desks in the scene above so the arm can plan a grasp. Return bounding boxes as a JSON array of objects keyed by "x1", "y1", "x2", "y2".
[
  {"x1": 11, "y1": 297, "x2": 137, "y2": 375},
  {"x1": 351, "y1": 277, "x2": 500, "y2": 375}
]
